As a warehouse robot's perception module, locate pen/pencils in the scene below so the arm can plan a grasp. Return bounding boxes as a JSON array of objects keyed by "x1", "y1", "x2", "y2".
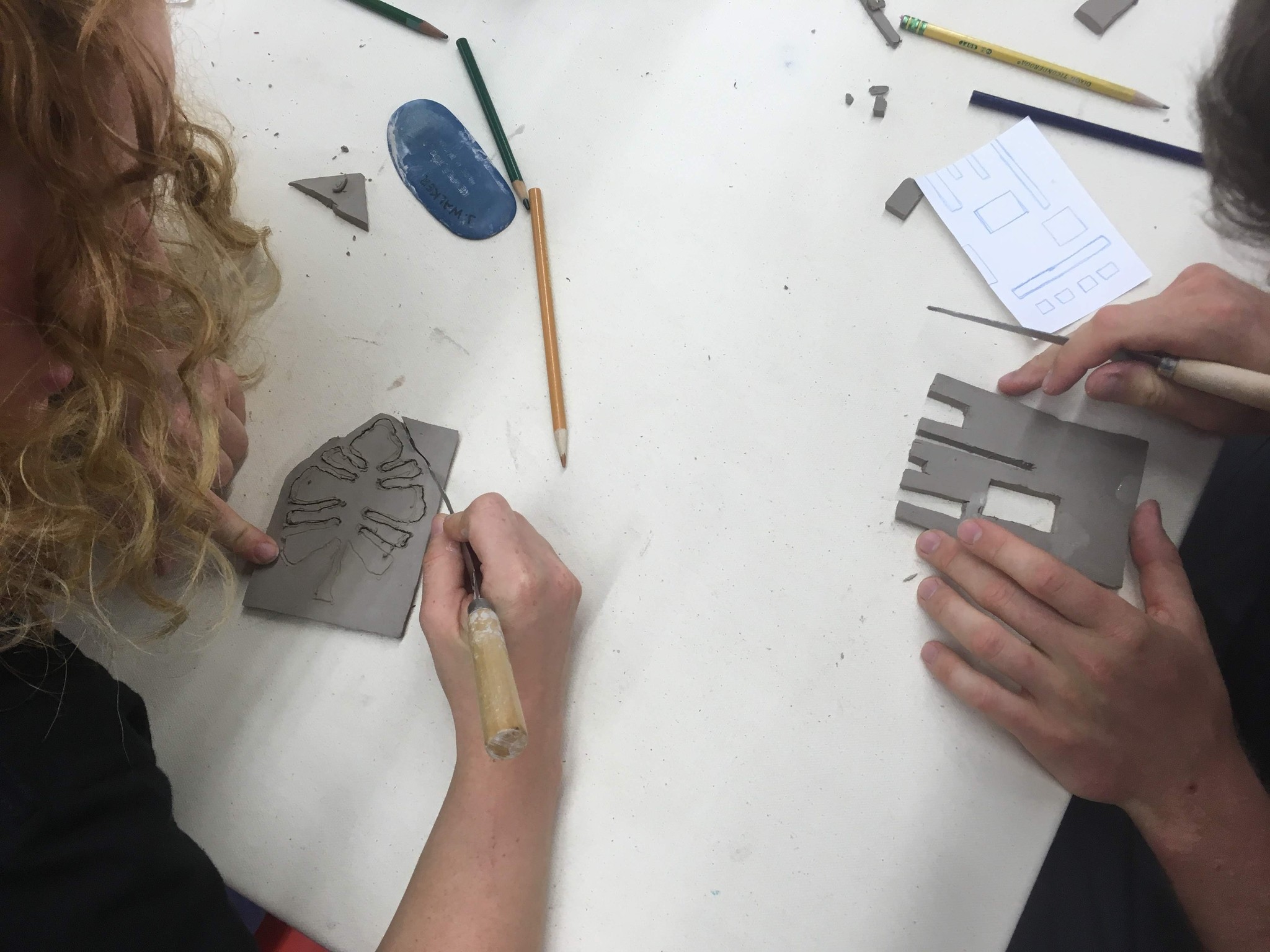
[
  {"x1": 530, "y1": 186, "x2": 569, "y2": 467},
  {"x1": 456, "y1": 36, "x2": 531, "y2": 214},
  {"x1": 899, "y1": 11, "x2": 1170, "y2": 110},
  {"x1": 347, "y1": 0, "x2": 450, "y2": 41},
  {"x1": 969, "y1": 88, "x2": 1205, "y2": 170}
]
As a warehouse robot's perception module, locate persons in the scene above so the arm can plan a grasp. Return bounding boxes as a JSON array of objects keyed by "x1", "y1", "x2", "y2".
[
  {"x1": 0, "y1": 0, "x2": 584, "y2": 952},
  {"x1": 911, "y1": 2, "x2": 1270, "y2": 951}
]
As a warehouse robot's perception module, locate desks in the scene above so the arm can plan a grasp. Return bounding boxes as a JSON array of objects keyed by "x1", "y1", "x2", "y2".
[{"x1": 44, "y1": 0, "x2": 1270, "y2": 952}]
